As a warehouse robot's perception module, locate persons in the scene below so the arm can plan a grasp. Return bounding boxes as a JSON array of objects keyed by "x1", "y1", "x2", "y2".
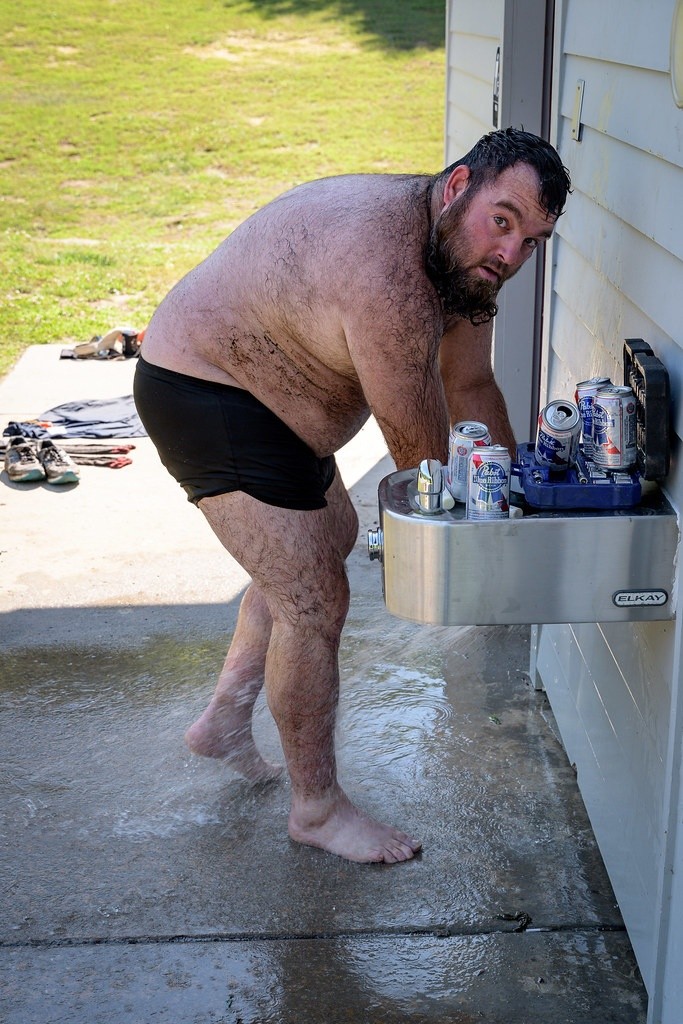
[{"x1": 133, "y1": 126, "x2": 571, "y2": 867}]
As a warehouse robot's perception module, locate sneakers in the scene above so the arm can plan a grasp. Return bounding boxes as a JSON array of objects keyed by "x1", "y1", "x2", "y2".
[
  {"x1": 36, "y1": 439, "x2": 81, "y2": 484},
  {"x1": 4, "y1": 436, "x2": 46, "y2": 483}
]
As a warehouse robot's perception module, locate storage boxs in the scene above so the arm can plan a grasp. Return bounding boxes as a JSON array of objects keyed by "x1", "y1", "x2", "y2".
[{"x1": 514, "y1": 338, "x2": 670, "y2": 511}]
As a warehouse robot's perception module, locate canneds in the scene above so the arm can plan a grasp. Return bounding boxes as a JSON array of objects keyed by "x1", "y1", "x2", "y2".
[
  {"x1": 535, "y1": 378, "x2": 638, "y2": 470},
  {"x1": 447, "y1": 420, "x2": 511, "y2": 519}
]
